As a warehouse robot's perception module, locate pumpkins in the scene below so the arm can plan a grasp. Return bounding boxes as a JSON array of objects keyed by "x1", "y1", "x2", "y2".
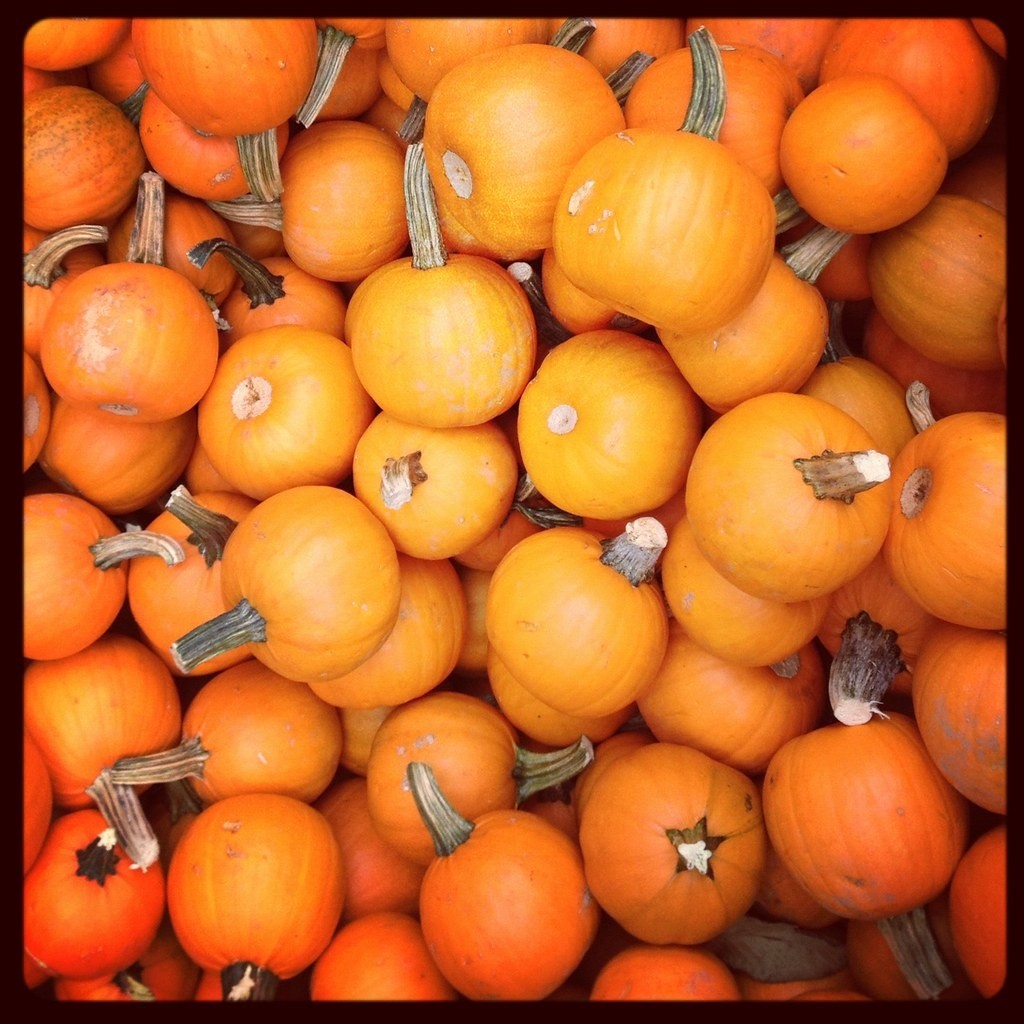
[{"x1": 24, "y1": 19, "x2": 1005, "y2": 1000}]
